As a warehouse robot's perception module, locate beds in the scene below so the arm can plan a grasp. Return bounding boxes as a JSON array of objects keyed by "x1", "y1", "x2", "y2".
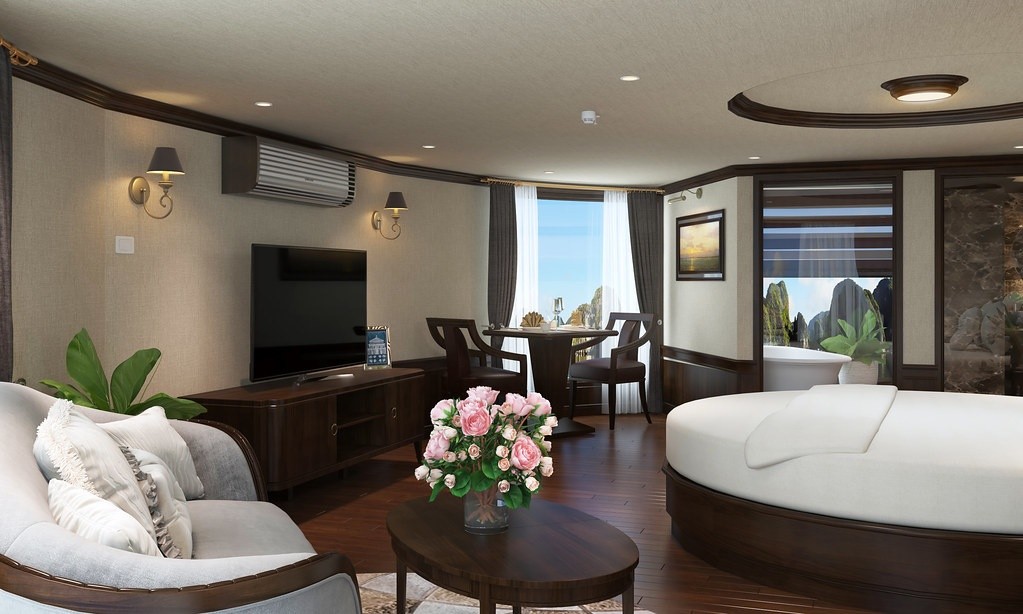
[{"x1": 661, "y1": 390, "x2": 1023, "y2": 614}]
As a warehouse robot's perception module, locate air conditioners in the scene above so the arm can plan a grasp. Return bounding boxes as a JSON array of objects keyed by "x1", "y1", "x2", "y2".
[{"x1": 221, "y1": 135, "x2": 356, "y2": 208}]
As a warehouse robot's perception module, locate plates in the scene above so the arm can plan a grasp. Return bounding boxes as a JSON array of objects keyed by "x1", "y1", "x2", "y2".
[{"x1": 518, "y1": 325, "x2": 540, "y2": 330}]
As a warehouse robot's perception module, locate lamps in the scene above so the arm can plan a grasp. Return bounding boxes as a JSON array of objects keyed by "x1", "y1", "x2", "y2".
[
  {"x1": 668, "y1": 188, "x2": 701, "y2": 204},
  {"x1": 372, "y1": 192, "x2": 408, "y2": 241},
  {"x1": 129, "y1": 147, "x2": 185, "y2": 219},
  {"x1": 881, "y1": 76, "x2": 968, "y2": 102}
]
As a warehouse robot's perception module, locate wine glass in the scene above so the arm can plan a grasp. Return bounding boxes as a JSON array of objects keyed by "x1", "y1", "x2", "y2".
[{"x1": 553, "y1": 299, "x2": 561, "y2": 323}]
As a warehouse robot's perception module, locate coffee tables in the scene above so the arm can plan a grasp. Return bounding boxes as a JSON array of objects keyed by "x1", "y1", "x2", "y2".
[{"x1": 386, "y1": 494, "x2": 639, "y2": 614}]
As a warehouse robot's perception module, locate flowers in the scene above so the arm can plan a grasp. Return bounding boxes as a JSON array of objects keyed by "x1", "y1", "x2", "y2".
[{"x1": 415, "y1": 387, "x2": 558, "y2": 509}]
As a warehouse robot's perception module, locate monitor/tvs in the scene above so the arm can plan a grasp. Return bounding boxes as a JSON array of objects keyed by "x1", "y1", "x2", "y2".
[{"x1": 249, "y1": 243, "x2": 369, "y2": 385}]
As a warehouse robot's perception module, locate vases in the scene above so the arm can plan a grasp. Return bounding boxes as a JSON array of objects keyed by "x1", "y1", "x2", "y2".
[{"x1": 463, "y1": 482, "x2": 509, "y2": 530}]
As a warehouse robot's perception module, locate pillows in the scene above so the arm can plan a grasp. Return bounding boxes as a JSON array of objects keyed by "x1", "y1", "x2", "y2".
[
  {"x1": 97, "y1": 405, "x2": 204, "y2": 501},
  {"x1": 34, "y1": 399, "x2": 157, "y2": 542},
  {"x1": 48, "y1": 480, "x2": 165, "y2": 557},
  {"x1": 119, "y1": 444, "x2": 192, "y2": 560}
]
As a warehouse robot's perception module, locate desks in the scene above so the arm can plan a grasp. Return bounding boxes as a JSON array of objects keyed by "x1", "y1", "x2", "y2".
[{"x1": 480, "y1": 329, "x2": 618, "y2": 440}]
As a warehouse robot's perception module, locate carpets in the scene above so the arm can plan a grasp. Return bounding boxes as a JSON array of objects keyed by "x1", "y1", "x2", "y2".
[{"x1": 357, "y1": 572, "x2": 657, "y2": 614}]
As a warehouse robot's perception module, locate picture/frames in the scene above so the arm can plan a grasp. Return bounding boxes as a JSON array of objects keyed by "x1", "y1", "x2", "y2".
[
  {"x1": 933, "y1": 168, "x2": 1023, "y2": 396},
  {"x1": 675, "y1": 209, "x2": 725, "y2": 282}
]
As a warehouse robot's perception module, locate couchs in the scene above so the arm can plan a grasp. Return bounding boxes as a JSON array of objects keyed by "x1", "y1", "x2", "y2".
[{"x1": 0, "y1": 389, "x2": 354, "y2": 614}]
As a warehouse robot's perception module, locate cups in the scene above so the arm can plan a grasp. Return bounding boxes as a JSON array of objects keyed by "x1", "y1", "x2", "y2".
[{"x1": 541, "y1": 323, "x2": 551, "y2": 331}]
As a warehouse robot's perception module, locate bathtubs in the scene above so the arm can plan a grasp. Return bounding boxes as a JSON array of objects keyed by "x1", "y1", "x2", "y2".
[{"x1": 763, "y1": 345, "x2": 852, "y2": 391}]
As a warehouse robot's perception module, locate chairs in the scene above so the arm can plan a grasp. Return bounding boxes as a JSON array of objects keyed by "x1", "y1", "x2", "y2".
[
  {"x1": 570, "y1": 312, "x2": 655, "y2": 431},
  {"x1": 424, "y1": 318, "x2": 528, "y2": 433}
]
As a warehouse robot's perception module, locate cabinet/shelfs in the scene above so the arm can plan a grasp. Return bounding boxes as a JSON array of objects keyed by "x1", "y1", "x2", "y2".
[{"x1": 178, "y1": 365, "x2": 431, "y2": 501}]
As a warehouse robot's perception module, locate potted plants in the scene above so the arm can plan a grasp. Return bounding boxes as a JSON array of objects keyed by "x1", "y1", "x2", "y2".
[{"x1": 821, "y1": 310, "x2": 890, "y2": 384}]
